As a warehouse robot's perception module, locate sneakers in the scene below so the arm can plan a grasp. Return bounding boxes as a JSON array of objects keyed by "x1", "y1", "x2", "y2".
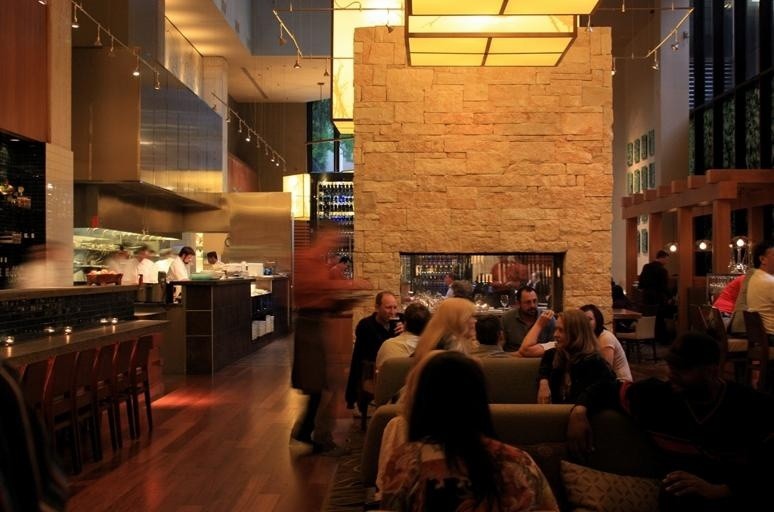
[{"x1": 291, "y1": 428, "x2": 352, "y2": 457}]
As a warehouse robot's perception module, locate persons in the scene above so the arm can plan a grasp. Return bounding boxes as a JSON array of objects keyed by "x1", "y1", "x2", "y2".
[
  {"x1": 640, "y1": 251, "x2": 677, "y2": 315},
  {"x1": 208, "y1": 251, "x2": 225, "y2": 278},
  {"x1": 560, "y1": 332, "x2": 774, "y2": 511},
  {"x1": 612, "y1": 286, "x2": 632, "y2": 332},
  {"x1": 372, "y1": 351, "x2": 559, "y2": 510},
  {"x1": 731, "y1": 241, "x2": 774, "y2": 345},
  {"x1": 121, "y1": 245, "x2": 158, "y2": 285},
  {"x1": 708, "y1": 276, "x2": 745, "y2": 331},
  {"x1": 290, "y1": 220, "x2": 375, "y2": 455},
  {"x1": 344, "y1": 271, "x2": 632, "y2": 454},
  {"x1": 166, "y1": 247, "x2": 194, "y2": 282}
]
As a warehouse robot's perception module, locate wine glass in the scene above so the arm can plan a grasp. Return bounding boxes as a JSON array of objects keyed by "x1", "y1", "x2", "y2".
[{"x1": 402, "y1": 290, "x2": 510, "y2": 313}]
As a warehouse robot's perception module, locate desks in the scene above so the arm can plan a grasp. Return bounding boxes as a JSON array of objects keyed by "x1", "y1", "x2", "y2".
[{"x1": 0, "y1": 319, "x2": 169, "y2": 403}]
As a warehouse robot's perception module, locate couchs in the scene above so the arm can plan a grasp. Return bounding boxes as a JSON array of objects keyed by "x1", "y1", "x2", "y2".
[
  {"x1": 374, "y1": 358, "x2": 542, "y2": 403},
  {"x1": 361, "y1": 403, "x2": 649, "y2": 484}
]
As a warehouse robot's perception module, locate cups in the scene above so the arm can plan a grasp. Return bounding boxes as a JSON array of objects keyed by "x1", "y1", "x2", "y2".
[{"x1": 388, "y1": 315, "x2": 400, "y2": 334}]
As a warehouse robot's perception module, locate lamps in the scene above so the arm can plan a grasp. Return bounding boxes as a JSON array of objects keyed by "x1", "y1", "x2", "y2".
[
  {"x1": 585, "y1": 15, "x2": 593, "y2": 32},
  {"x1": 724, "y1": 0, "x2": 734, "y2": 10},
  {"x1": 386, "y1": 25, "x2": 393, "y2": 33},
  {"x1": 671, "y1": 29, "x2": 679, "y2": 51},
  {"x1": 70, "y1": 2, "x2": 303, "y2": 169},
  {"x1": 651, "y1": 51, "x2": 660, "y2": 71}
]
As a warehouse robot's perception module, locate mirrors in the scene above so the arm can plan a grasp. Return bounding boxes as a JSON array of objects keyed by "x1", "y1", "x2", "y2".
[{"x1": 401, "y1": 250, "x2": 568, "y2": 307}]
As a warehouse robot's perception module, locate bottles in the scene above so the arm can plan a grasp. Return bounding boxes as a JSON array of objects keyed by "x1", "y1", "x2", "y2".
[
  {"x1": 537, "y1": 378, "x2": 552, "y2": 404},
  {"x1": 137, "y1": 275, "x2": 146, "y2": 302},
  {"x1": 0, "y1": 256, "x2": 17, "y2": 288},
  {"x1": 415, "y1": 256, "x2": 493, "y2": 298},
  {"x1": 241, "y1": 260, "x2": 247, "y2": 279},
  {"x1": 319, "y1": 183, "x2": 354, "y2": 279}
]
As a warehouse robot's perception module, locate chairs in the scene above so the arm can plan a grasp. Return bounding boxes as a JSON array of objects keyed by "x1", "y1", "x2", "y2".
[
  {"x1": 46, "y1": 352, "x2": 80, "y2": 474},
  {"x1": 114, "y1": 340, "x2": 137, "y2": 449},
  {"x1": 703, "y1": 304, "x2": 748, "y2": 378},
  {"x1": 21, "y1": 360, "x2": 50, "y2": 441},
  {"x1": 742, "y1": 310, "x2": 774, "y2": 383},
  {"x1": 73, "y1": 348, "x2": 98, "y2": 471},
  {"x1": 689, "y1": 303, "x2": 708, "y2": 334},
  {"x1": 93, "y1": 341, "x2": 121, "y2": 459},
  {"x1": 617, "y1": 316, "x2": 658, "y2": 364},
  {"x1": 131, "y1": 334, "x2": 154, "y2": 437}
]
